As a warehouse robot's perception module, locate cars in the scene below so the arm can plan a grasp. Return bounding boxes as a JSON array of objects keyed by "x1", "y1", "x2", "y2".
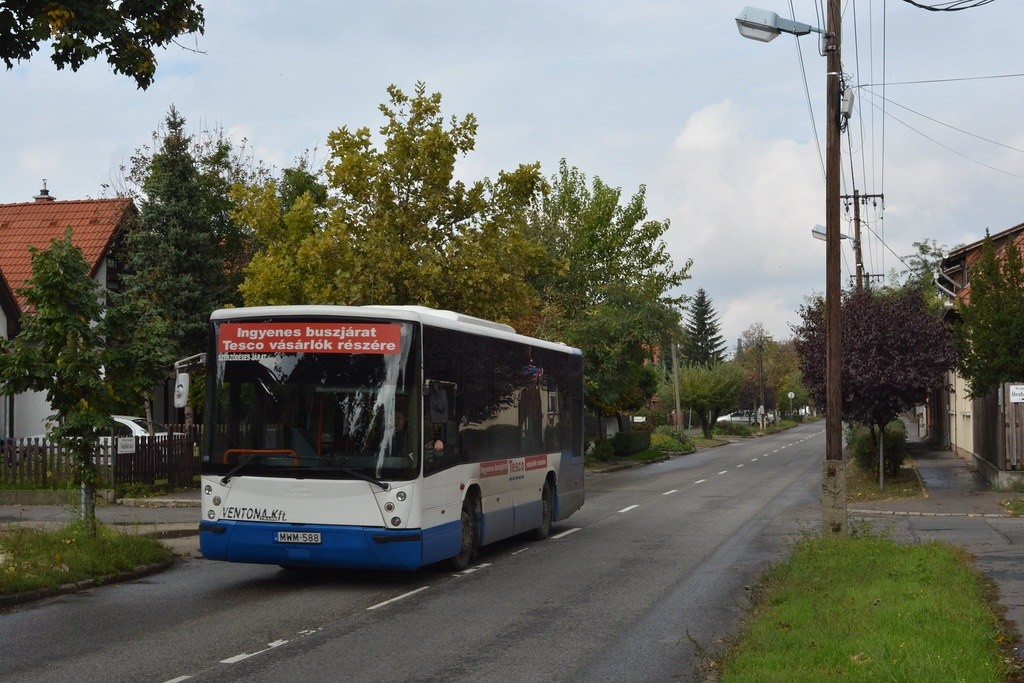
[
  {"x1": 15, "y1": 415, "x2": 185, "y2": 466},
  {"x1": 766, "y1": 410, "x2": 781, "y2": 423},
  {"x1": 718, "y1": 409, "x2": 770, "y2": 427}
]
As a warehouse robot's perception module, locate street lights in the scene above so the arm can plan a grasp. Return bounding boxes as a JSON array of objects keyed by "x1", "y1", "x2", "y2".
[
  {"x1": 811, "y1": 224, "x2": 864, "y2": 290},
  {"x1": 735, "y1": 5, "x2": 848, "y2": 461}
]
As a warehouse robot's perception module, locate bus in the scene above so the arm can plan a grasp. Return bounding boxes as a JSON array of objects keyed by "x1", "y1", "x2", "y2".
[{"x1": 174, "y1": 304, "x2": 584, "y2": 574}]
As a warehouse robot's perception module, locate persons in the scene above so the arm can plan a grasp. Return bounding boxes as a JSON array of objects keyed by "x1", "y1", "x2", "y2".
[{"x1": 363, "y1": 406, "x2": 444, "y2": 456}]
